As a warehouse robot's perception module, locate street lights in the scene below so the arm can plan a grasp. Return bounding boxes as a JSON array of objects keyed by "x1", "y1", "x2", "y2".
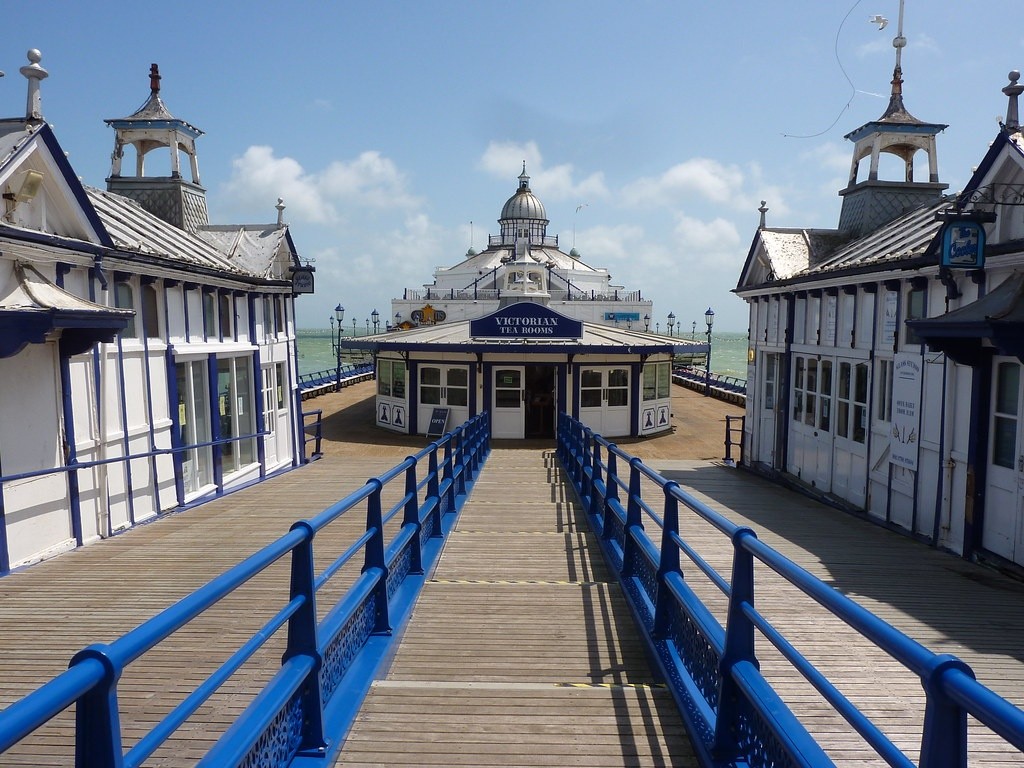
[
  {"x1": 704, "y1": 306, "x2": 715, "y2": 397},
  {"x1": 352, "y1": 317, "x2": 357, "y2": 336},
  {"x1": 366, "y1": 318, "x2": 370, "y2": 335},
  {"x1": 689, "y1": 321, "x2": 697, "y2": 368},
  {"x1": 376, "y1": 319, "x2": 380, "y2": 334},
  {"x1": 667, "y1": 312, "x2": 675, "y2": 337},
  {"x1": 643, "y1": 313, "x2": 651, "y2": 333},
  {"x1": 330, "y1": 316, "x2": 335, "y2": 357},
  {"x1": 655, "y1": 323, "x2": 659, "y2": 335},
  {"x1": 370, "y1": 308, "x2": 380, "y2": 335},
  {"x1": 677, "y1": 321, "x2": 681, "y2": 337},
  {"x1": 335, "y1": 303, "x2": 345, "y2": 393}
]
